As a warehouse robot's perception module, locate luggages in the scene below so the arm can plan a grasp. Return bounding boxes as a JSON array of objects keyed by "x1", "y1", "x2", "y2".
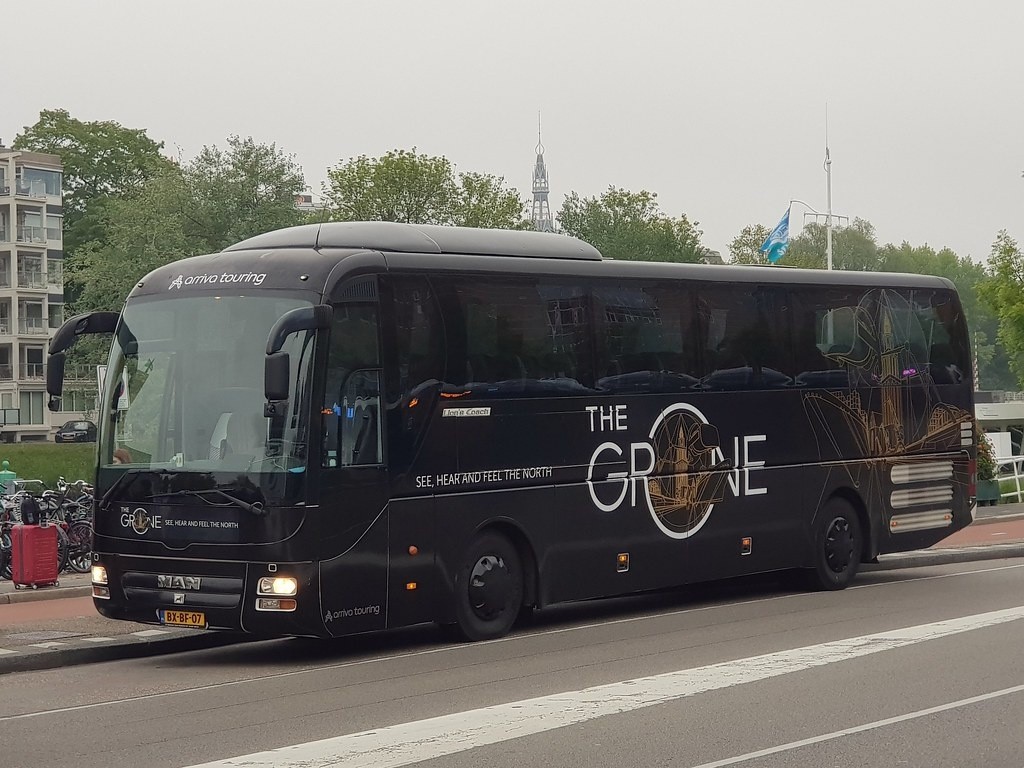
[{"x1": 11, "y1": 495, "x2": 60, "y2": 590}]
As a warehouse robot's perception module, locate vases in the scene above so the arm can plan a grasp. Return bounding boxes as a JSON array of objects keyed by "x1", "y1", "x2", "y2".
[{"x1": 975, "y1": 481, "x2": 1001, "y2": 507}]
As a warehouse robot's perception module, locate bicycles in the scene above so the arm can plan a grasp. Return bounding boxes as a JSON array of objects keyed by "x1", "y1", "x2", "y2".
[{"x1": 0, "y1": 474, "x2": 94, "y2": 582}]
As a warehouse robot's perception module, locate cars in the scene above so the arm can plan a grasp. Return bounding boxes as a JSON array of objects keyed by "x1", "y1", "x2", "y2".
[{"x1": 54, "y1": 421, "x2": 97, "y2": 443}]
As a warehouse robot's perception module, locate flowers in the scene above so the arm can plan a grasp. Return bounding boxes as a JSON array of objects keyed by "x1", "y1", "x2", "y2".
[{"x1": 976, "y1": 420, "x2": 998, "y2": 479}]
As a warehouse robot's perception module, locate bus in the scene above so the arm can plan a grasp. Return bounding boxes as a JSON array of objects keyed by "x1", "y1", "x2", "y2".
[{"x1": 43, "y1": 219, "x2": 980, "y2": 642}]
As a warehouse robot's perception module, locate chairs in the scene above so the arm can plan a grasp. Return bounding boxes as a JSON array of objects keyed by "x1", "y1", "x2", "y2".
[{"x1": 985, "y1": 432, "x2": 1024, "y2": 503}]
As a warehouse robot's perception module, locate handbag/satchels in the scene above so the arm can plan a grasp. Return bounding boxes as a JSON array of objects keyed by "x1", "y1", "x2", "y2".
[{"x1": 21, "y1": 497, "x2": 40, "y2": 525}]
